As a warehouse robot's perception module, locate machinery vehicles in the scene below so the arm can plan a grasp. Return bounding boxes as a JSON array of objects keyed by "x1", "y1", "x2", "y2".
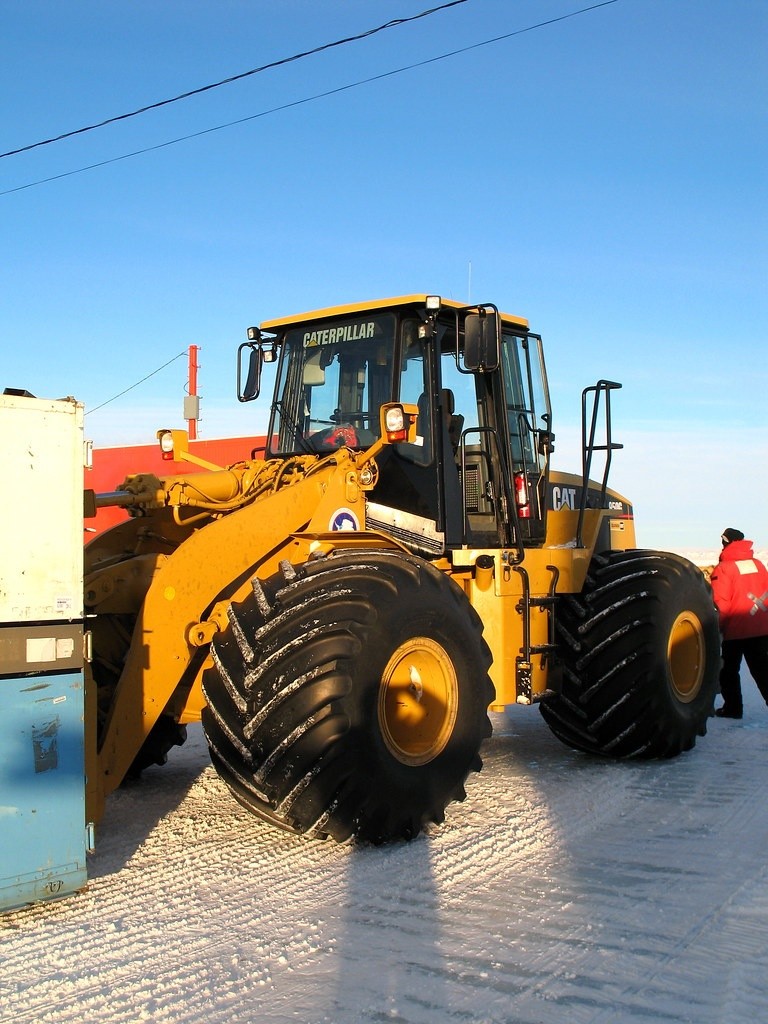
[{"x1": 83, "y1": 292, "x2": 726, "y2": 849}]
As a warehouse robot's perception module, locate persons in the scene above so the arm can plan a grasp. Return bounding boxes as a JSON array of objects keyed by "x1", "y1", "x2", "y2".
[{"x1": 710, "y1": 528, "x2": 768, "y2": 721}]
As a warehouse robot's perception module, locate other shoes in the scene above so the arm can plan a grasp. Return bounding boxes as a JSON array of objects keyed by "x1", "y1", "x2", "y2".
[{"x1": 716, "y1": 708, "x2": 742, "y2": 719}]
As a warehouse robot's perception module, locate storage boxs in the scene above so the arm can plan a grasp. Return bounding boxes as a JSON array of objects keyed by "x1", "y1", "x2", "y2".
[
  {"x1": 0, "y1": 673, "x2": 89, "y2": 914},
  {"x1": 0, "y1": 393, "x2": 85, "y2": 624}
]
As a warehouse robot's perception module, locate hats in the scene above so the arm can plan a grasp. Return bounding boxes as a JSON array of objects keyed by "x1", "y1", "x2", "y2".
[{"x1": 721, "y1": 528, "x2": 744, "y2": 545}]
